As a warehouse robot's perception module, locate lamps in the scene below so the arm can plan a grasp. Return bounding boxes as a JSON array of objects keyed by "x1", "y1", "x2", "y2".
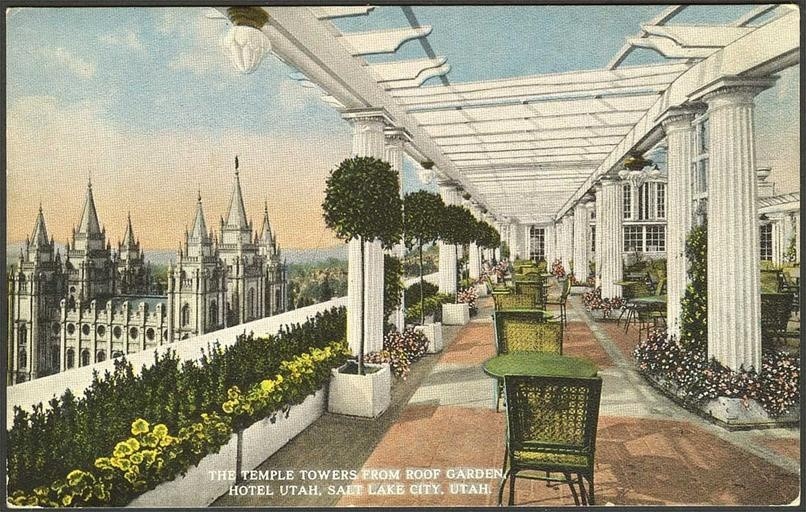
[{"x1": 223, "y1": 6, "x2": 272, "y2": 75}]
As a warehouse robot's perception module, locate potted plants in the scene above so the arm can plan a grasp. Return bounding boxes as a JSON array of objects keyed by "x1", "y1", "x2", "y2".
[
  {"x1": 401, "y1": 188, "x2": 448, "y2": 354},
  {"x1": 323, "y1": 154, "x2": 402, "y2": 419},
  {"x1": 6, "y1": 335, "x2": 352, "y2": 511},
  {"x1": 444, "y1": 205, "x2": 500, "y2": 325}
]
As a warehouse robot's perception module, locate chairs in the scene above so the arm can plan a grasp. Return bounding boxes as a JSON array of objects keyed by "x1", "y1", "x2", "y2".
[
  {"x1": 483, "y1": 260, "x2": 605, "y2": 506},
  {"x1": 615, "y1": 265, "x2": 801, "y2": 347}
]
